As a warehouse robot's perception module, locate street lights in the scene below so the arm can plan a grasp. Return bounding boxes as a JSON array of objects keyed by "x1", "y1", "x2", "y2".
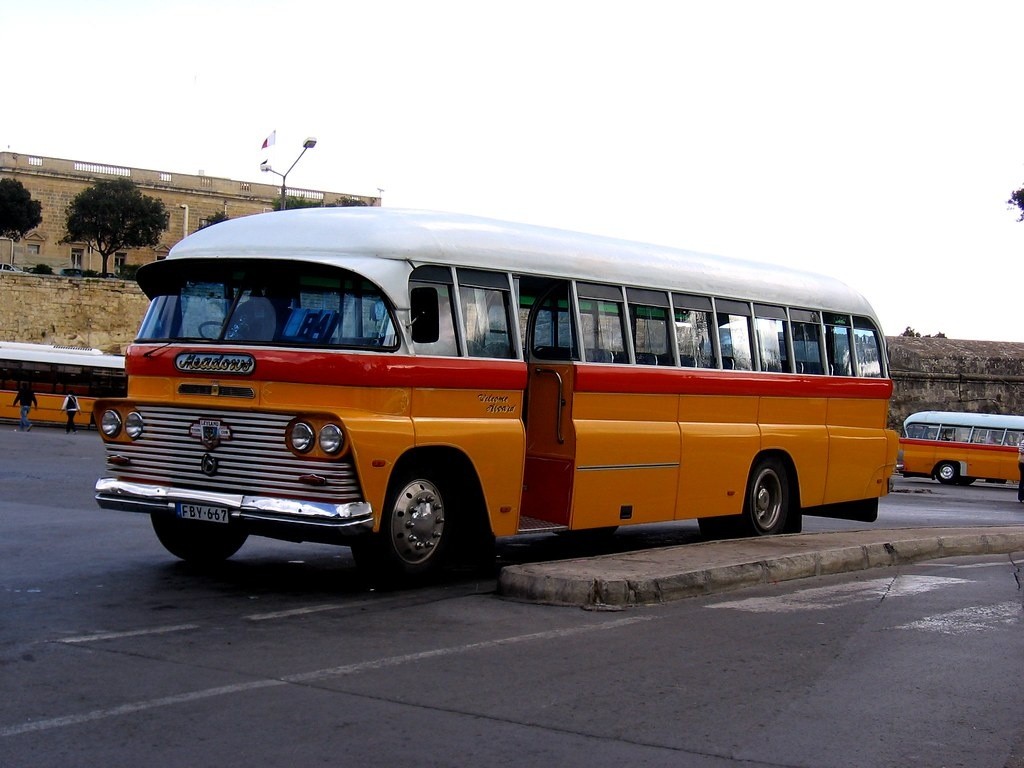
[{"x1": 261, "y1": 136, "x2": 318, "y2": 210}]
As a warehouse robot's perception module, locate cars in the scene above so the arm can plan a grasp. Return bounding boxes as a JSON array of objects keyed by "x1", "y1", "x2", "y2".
[
  {"x1": 61, "y1": 268, "x2": 83, "y2": 277},
  {"x1": 97, "y1": 273, "x2": 119, "y2": 278},
  {"x1": 0, "y1": 263, "x2": 29, "y2": 274}
]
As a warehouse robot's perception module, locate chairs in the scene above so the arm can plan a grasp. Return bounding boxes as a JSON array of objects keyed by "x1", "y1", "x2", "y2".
[
  {"x1": 487, "y1": 339, "x2": 845, "y2": 376},
  {"x1": 218, "y1": 297, "x2": 278, "y2": 344}
]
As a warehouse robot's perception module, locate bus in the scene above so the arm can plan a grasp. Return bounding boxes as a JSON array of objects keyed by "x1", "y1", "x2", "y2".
[
  {"x1": 94, "y1": 206, "x2": 897, "y2": 595},
  {"x1": 898, "y1": 411, "x2": 1024, "y2": 487},
  {"x1": 0, "y1": 339, "x2": 126, "y2": 429}
]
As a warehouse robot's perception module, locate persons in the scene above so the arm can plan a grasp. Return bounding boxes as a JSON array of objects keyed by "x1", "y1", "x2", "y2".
[
  {"x1": 1017, "y1": 440, "x2": 1024, "y2": 503},
  {"x1": 59, "y1": 390, "x2": 82, "y2": 435},
  {"x1": 12, "y1": 382, "x2": 38, "y2": 432},
  {"x1": 985, "y1": 431, "x2": 1015, "y2": 447},
  {"x1": 238, "y1": 286, "x2": 280, "y2": 342}
]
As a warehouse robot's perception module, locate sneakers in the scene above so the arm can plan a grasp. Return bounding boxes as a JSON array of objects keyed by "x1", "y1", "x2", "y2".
[
  {"x1": 64, "y1": 430, "x2": 68, "y2": 433},
  {"x1": 26, "y1": 424, "x2": 32, "y2": 431},
  {"x1": 72, "y1": 431, "x2": 77, "y2": 433}
]
